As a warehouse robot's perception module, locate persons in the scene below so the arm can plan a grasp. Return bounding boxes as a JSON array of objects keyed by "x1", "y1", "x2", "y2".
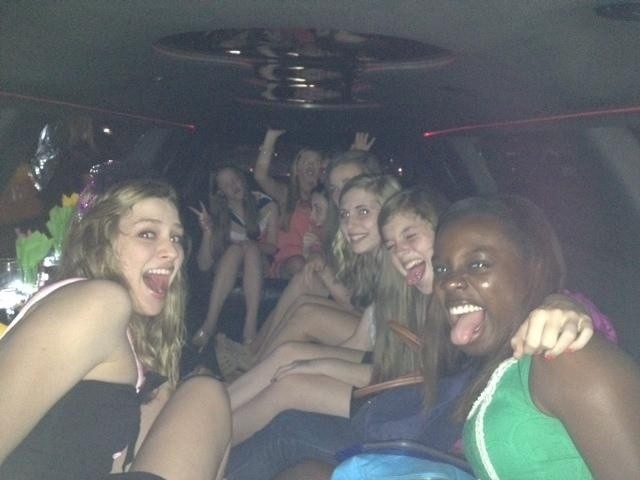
[{"x1": 1, "y1": 129, "x2": 639, "y2": 480}]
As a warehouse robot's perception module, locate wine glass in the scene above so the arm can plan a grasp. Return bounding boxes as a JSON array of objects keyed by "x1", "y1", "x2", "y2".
[{"x1": 0, "y1": 257, "x2": 22, "y2": 326}]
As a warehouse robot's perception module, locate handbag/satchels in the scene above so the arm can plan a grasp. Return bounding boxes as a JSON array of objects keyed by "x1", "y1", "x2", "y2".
[{"x1": 347, "y1": 322, "x2": 475, "y2": 445}]
